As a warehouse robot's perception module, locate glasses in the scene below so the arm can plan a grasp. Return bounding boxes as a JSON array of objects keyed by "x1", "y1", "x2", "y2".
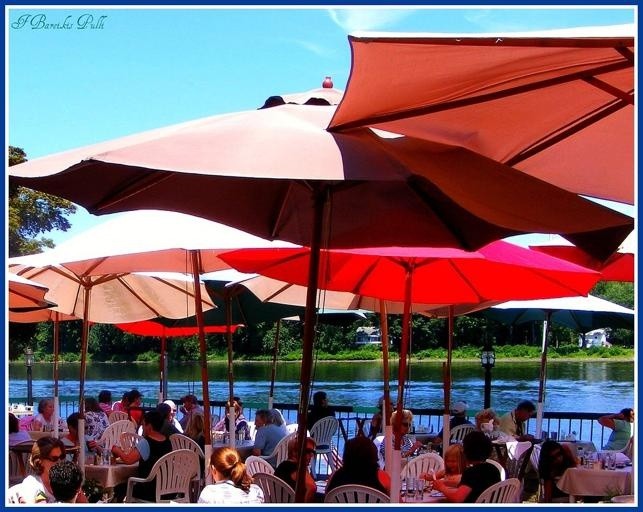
[
  {"x1": 402, "y1": 422, "x2": 411, "y2": 427},
  {"x1": 44, "y1": 454, "x2": 66, "y2": 462}
]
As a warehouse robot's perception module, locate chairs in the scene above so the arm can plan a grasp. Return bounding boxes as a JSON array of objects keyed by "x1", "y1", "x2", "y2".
[{"x1": 8, "y1": 399, "x2": 634, "y2": 504}]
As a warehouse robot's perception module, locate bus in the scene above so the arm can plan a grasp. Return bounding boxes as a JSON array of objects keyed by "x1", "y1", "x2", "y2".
[{"x1": 354, "y1": 333, "x2": 393, "y2": 351}]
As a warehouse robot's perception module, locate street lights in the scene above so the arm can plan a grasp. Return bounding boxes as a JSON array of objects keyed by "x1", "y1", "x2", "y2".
[
  {"x1": 23, "y1": 347, "x2": 37, "y2": 409},
  {"x1": 478, "y1": 336, "x2": 499, "y2": 415}
]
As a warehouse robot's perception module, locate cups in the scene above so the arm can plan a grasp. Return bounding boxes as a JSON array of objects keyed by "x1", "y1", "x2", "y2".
[
  {"x1": 416, "y1": 425, "x2": 428, "y2": 432},
  {"x1": 215, "y1": 426, "x2": 245, "y2": 445},
  {"x1": 44, "y1": 423, "x2": 51, "y2": 432},
  {"x1": 541, "y1": 431, "x2": 565, "y2": 441},
  {"x1": 401, "y1": 475, "x2": 424, "y2": 499},
  {"x1": 480, "y1": 423, "x2": 493, "y2": 432}
]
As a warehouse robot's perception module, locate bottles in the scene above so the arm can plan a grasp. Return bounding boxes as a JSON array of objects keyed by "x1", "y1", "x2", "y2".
[
  {"x1": 576, "y1": 447, "x2": 616, "y2": 469},
  {"x1": 88, "y1": 446, "x2": 111, "y2": 465},
  {"x1": 410, "y1": 420, "x2": 415, "y2": 433},
  {"x1": 421, "y1": 441, "x2": 432, "y2": 454}
]
